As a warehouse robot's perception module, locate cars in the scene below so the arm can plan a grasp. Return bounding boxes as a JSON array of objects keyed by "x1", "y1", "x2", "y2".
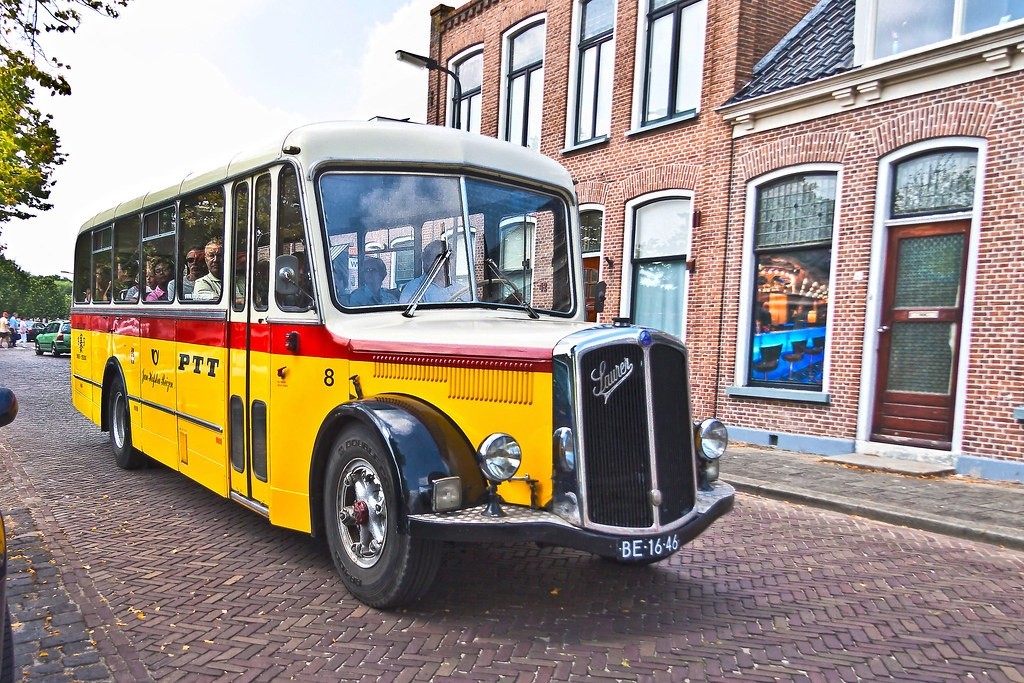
[
  {"x1": 24, "y1": 321, "x2": 47, "y2": 341},
  {"x1": 35, "y1": 321, "x2": 73, "y2": 357}
]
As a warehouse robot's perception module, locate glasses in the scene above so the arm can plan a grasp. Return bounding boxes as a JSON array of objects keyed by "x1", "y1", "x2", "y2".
[{"x1": 186, "y1": 258, "x2": 205, "y2": 265}]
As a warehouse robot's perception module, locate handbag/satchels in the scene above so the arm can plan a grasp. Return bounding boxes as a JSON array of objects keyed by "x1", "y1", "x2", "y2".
[{"x1": 13, "y1": 334, "x2": 21, "y2": 340}]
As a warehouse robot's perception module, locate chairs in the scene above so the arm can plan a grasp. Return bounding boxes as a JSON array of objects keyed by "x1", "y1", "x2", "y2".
[{"x1": 93, "y1": 280, "x2": 441, "y2": 310}]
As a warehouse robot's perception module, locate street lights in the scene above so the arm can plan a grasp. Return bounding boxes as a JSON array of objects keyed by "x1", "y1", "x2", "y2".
[{"x1": 393, "y1": 48, "x2": 463, "y2": 132}]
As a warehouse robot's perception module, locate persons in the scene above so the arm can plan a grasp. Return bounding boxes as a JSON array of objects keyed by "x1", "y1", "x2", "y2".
[
  {"x1": 86, "y1": 240, "x2": 316, "y2": 308},
  {"x1": 348, "y1": 257, "x2": 399, "y2": 304},
  {"x1": 16, "y1": 316, "x2": 28, "y2": 347},
  {"x1": 9, "y1": 311, "x2": 18, "y2": 347},
  {"x1": 0, "y1": 311, "x2": 13, "y2": 348},
  {"x1": 399, "y1": 240, "x2": 473, "y2": 303}
]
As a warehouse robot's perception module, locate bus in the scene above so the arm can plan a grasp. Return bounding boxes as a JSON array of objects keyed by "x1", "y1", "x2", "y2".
[{"x1": 69, "y1": 115, "x2": 740, "y2": 609}]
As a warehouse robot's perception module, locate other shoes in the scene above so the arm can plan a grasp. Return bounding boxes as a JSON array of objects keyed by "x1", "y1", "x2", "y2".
[
  {"x1": 0, "y1": 345, "x2": 4, "y2": 348},
  {"x1": 8, "y1": 345, "x2": 13, "y2": 348},
  {"x1": 22, "y1": 346, "x2": 27, "y2": 348}
]
